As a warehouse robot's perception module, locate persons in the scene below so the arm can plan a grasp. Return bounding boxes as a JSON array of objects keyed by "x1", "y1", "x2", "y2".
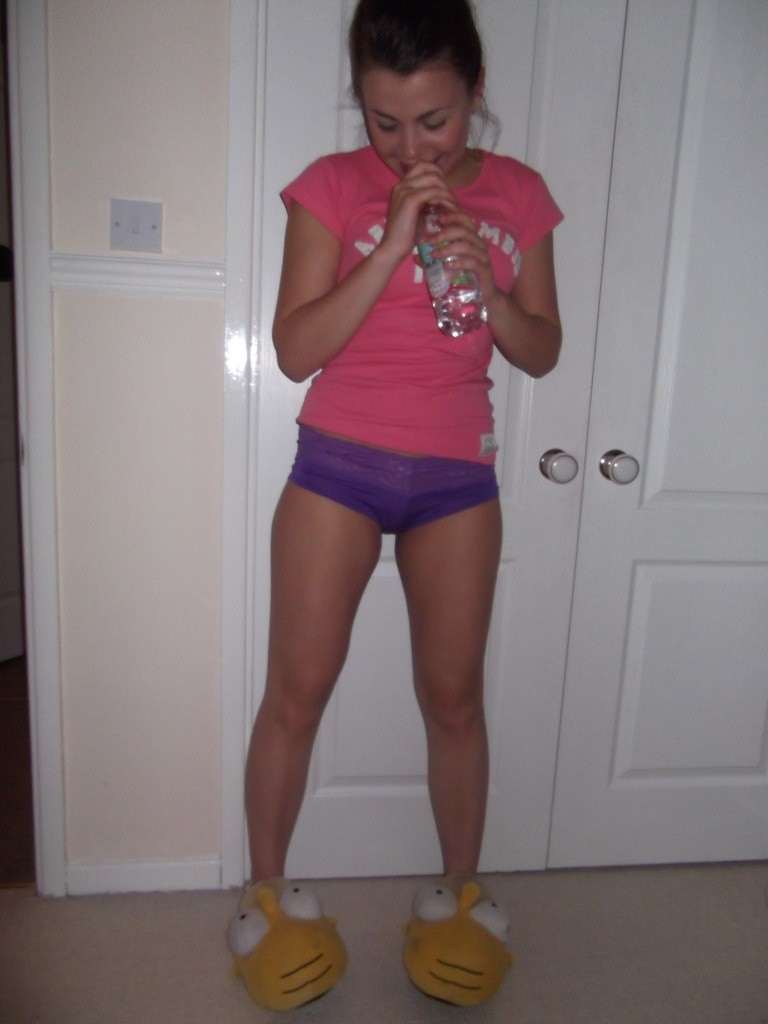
[{"x1": 225, "y1": 2, "x2": 566, "y2": 1010}]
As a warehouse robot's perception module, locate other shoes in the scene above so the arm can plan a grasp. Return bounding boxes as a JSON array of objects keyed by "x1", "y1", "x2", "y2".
[
  {"x1": 403, "y1": 874, "x2": 515, "y2": 1007},
  {"x1": 224, "y1": 873, "x2": 350, "y2": 1012}
]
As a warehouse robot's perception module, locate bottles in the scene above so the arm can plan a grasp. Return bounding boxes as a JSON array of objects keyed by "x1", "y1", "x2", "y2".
[{"x1": 415, "y1": 202, "x2": 490, "y2": 337}]
